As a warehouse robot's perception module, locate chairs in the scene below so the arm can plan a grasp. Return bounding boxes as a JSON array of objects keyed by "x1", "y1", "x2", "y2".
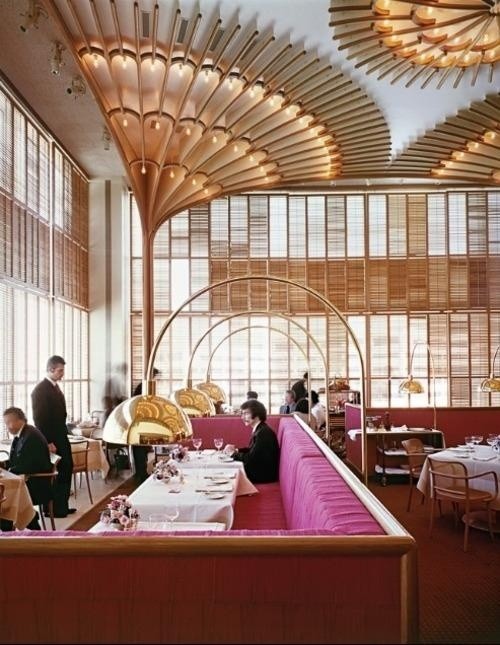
[
  {"x1": 427, "y1": 458, "x2": 498, "y2": 553},
  {"x1": 12, "y1": 452, "x2": 62, "y2": 531},
  {"x1": 70, "y1": 440, "x2": 93, "y2": 504},
  {"x1": 400, "y1": 438, "x2": 426, "y2": 512}
]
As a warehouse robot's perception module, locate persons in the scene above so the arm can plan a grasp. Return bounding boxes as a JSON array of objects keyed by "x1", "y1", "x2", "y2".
[
  {"x1": 31, "y1": 355, "x2": 78, "y2": 518},
  {"x1": 244, "y1": 391, "x2": 259, "y2": 401},
  {"x1": 279, "y1": 390, "x2": 297, "y2": 415},
  {"x1": 291, "y1": 401, "x2": 315, "y2": 433},
  {"x1": 99, "y1": 358, "x2": 129, "y2": 482},
  {"x1": 309, "y1": 390, "x2": 326, "y2": 430},
  {"x1": 1, "y1": 407, "x2": 55, "y2": 532},
  {"x1": 222, "y1": 400, "x2": 281, "y2": 485},
  {"x1": 134, "y1": 367, "x2": 159, "y2": 394},
  {"x1": 291, "y1": 371, "x2": 308, "y2": 400}
]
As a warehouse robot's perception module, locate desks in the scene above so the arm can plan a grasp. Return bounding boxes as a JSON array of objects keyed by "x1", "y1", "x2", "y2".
[{"x1": 0, "y1": 467, "x2": 36, "y2": 531}]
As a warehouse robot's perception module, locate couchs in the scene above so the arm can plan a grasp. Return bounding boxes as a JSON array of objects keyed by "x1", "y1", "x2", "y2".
[{"x1": 0, "y1": 413, "x2": 420, "y2": 644}]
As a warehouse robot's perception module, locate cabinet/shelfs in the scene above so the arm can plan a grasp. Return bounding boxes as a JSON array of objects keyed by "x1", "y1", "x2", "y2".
[
  {"x1": 317, "y1": 388, "x2": 360, "y2": 447},
  {"x1": 366, "y1": 424, "x2": 446, "y2": 486}
]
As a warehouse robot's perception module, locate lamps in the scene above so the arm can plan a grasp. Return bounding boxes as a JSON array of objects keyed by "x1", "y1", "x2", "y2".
[
  {"x1": 398, "y1": 343, "x2": 436, "y2": 430},
  {"x1": 478, "y1": 345, "x2": 500, "y2": 393}
]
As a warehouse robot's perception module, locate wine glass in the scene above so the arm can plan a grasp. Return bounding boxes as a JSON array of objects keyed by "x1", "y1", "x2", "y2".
[
  {"x1": 164, "y1": 506, "x2": 179, "y2": 530},
  {"x1": 464, "y1": 434, "x2": 500, "y2": 452},
  {"x1": 213, "y1": 438, "x2": 223, "y2": 454},
  {"x1": 192, "y1": 438, "x2": 202, "y2": 455}
]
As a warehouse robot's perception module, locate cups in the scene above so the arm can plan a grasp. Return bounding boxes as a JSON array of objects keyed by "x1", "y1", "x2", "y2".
[{"x1": 148, "y1": 514, "x2": 166, "y2": 530}]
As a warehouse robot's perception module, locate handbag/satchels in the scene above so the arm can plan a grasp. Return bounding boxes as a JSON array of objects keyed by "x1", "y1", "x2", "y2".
[{"x1": 113, "y1": 448, "x2": 130, "y2": 470}]
